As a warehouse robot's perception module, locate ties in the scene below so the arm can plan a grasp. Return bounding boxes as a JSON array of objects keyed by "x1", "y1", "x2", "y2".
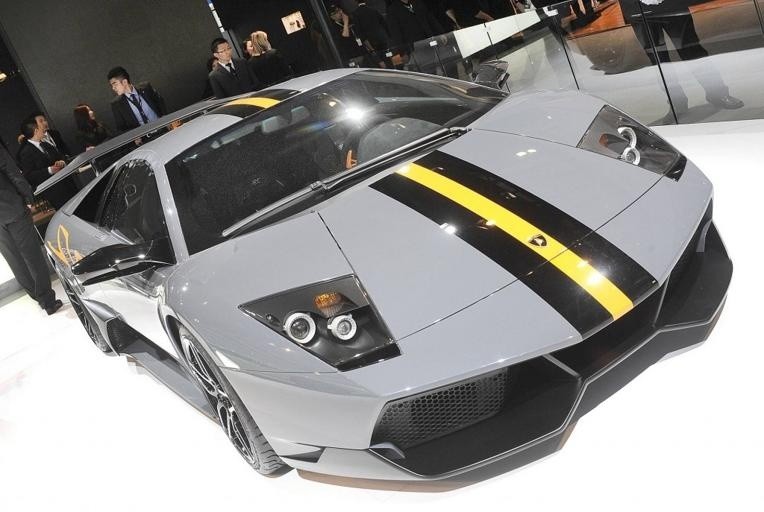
[
  {"x1": 130, "y1": 94, "x2": 148, "y2": 124},
  {"x1": 225, "y1": 63, "x2": 238, "y2": 79},
  {"x1": 40, "y1": 143, "x2": 46, "y2": 153}
]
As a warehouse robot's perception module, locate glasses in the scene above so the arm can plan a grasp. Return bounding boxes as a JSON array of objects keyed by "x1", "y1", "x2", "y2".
[
  {"x1": 330, "y1": 7, "x2": 341, "y2": 16},
  {"x1": 215, "y1": 47, "x2": 232, "y2": 53}
]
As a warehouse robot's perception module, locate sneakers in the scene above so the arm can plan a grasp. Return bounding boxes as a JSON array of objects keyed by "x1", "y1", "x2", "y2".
[{"x1": 45, "y1": 300, "x2": 62, "y2": 315}]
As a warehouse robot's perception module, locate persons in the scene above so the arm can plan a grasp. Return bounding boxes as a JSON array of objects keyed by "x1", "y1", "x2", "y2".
[
  {"x1": 70, "y1": 103, "x2": 124, "y2": 169},
  {"x1": 15, "y1": 110, "x2": 74, "y2": 167},
  {"x1": 242, "y1": 31, "x2": 291, "y2": 91},
  {"x1": 238, "y1": 38, "x2": 254, "y2": 64},
  {"x1": 0, "y1": 137, "x2": 63, "y2": 316},
  {"x1": 200, "y1": 57, "x2": 225, "y2": 100},
  {"x1": 325, "y1": 0, "x2": 605, "y2": 81},
  {"x1": 106, "y1": 65, "x2": 172, "y2": 148},
  {"x1": 205, "y1": 37, "x2": 260, "y2": 98},
  {"x1": 14, "y1": 117, "x2": 83, "y2": 209},
  {"x1": 616, "y1": 0, "x2": 745, "y2": 126}
]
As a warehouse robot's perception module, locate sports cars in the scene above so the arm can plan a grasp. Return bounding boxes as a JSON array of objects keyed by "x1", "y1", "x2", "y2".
[{"x1": 45, "y1": 62, "x2": 735, "y2": 498}]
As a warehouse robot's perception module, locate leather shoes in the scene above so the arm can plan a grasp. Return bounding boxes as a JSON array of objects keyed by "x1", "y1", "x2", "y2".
[
  {"x1": 662, "y1": 105, "x2": 689, "y2": 124},
  {"x1": 705, "y1": 91, "x2": 744, "y2": 109}
]
